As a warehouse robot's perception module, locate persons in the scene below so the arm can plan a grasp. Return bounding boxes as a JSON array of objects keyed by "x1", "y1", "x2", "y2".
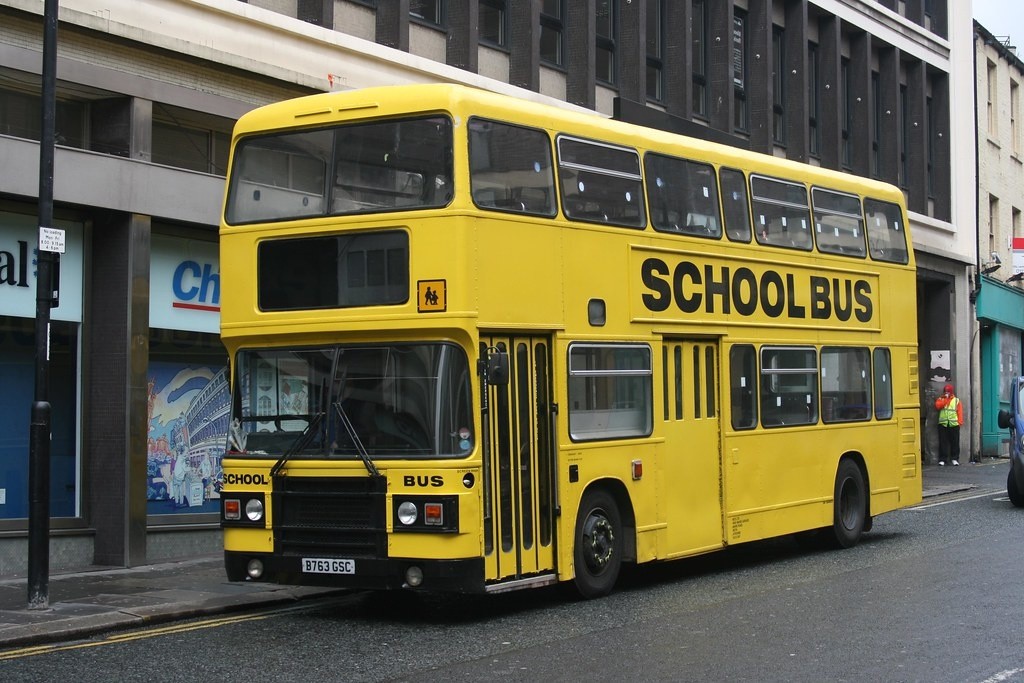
[{"x1": 935, "y1": 384, "x2": 964, "y2": 466}]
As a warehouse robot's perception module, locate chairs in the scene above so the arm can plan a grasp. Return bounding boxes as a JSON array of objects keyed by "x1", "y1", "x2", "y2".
[
  {"x1": 386, "y1": 171, "x2": 894, "y2": 262},
  {"x1": 732, "y1": 392, "x2": 885, "y2": 429}
]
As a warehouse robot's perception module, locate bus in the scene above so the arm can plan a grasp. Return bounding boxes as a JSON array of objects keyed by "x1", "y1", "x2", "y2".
[{"x1": 214, "y1": 82, "x2": 922, "y2": 604}]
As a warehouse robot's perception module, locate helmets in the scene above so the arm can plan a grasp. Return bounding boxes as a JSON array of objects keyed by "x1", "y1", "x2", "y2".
[{"x1": 944, "y1": 385, "x2": 954, "y2": 393}]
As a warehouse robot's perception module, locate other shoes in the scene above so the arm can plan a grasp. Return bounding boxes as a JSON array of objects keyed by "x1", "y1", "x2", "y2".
[
  {"x1": 952, "y1": 460, "x2": 959, "y2": 466},
  {"x1": 939, "y1": 461, "x2": 945, "y2": 465}
]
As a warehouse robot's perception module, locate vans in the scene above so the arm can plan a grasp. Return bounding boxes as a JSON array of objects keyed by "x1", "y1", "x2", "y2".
[{"x1": 998, "y1": 377, "x2": 1024, "y2": 506}]
